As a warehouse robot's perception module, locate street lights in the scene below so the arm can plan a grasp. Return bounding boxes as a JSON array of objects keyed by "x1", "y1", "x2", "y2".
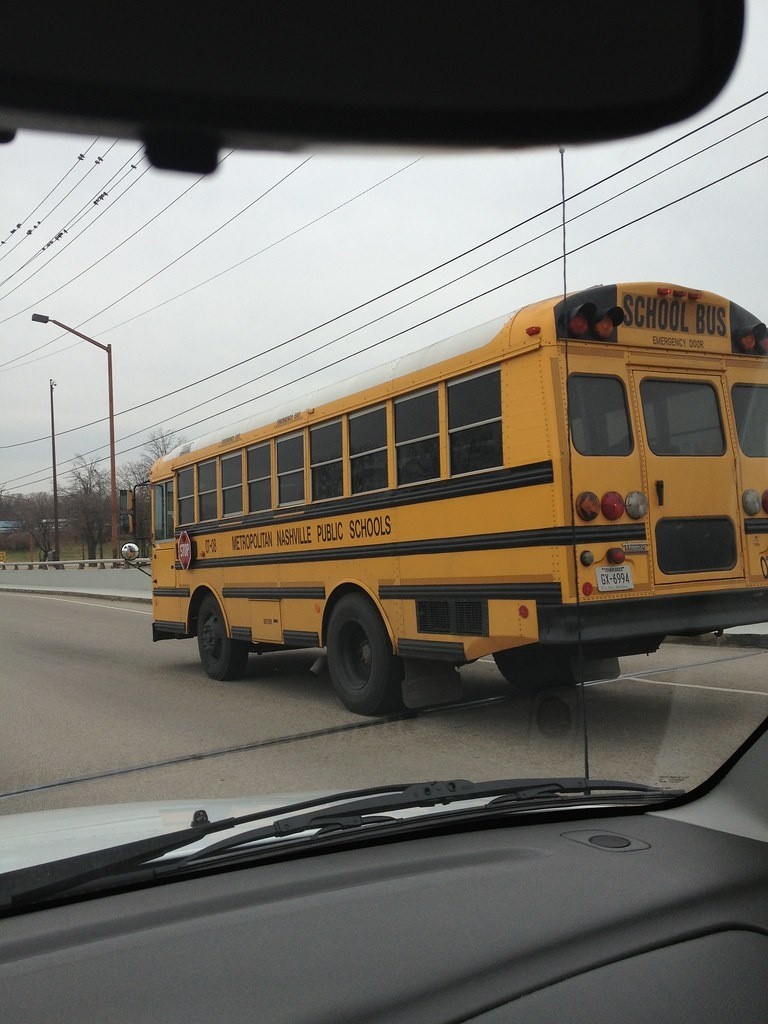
[{"x1": 31, "y1": 313, "x2": 117, "y2": 560}]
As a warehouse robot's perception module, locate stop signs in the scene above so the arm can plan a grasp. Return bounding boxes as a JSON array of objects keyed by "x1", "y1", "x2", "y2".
[{"x1": 177, "y1": 530, "x2": 192, "y2": 570}]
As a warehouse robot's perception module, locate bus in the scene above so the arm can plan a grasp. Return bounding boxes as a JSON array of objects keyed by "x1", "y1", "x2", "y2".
[{"x1": 121, "y1": 281, "x2": 768, "y2": 716}]
